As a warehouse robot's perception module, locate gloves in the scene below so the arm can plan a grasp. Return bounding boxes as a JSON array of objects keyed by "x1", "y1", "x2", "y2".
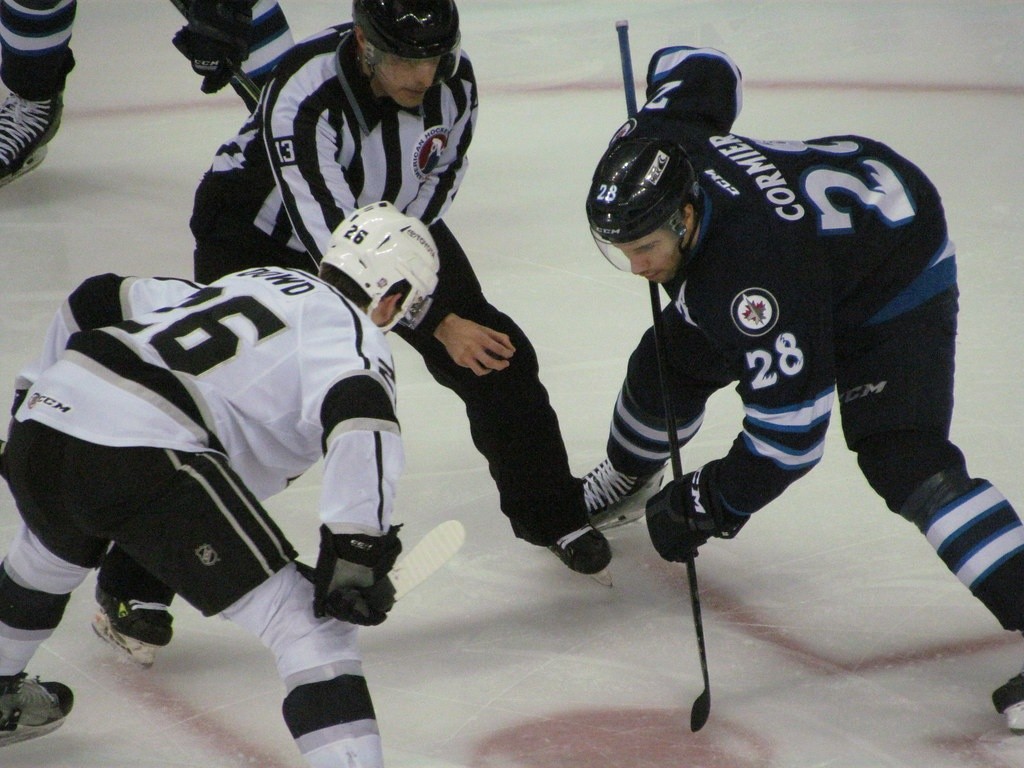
[
  {"x1": 645, "y1": 459, "x2": 750, "y2": 565},
  {"x1": 170, "y1": 0, "x2": 255, "y2": 95},
  {"x1": 312, "y1": 522, "x2": 404, "y2": 627}
]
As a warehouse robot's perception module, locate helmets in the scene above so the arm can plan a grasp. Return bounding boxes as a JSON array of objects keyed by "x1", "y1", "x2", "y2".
[
  {"x1": 316, "y1": 201, "x2": 440, "y2": 332},
  {"x1": 352, "y1": 0, "x2": 462, "y2": 57},
  {"x1": 586, "y1": 137, "x2": 705, "y2": 243}
]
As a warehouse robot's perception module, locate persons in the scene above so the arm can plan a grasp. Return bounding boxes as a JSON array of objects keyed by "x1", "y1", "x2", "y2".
[
  {"x1": 1, "y1": 1, "x2": 295, "y2": 187},
  {"x1": 94, "y1": 0, "x2": 615, "y2": 666},
  {"x1": 580, "y1": 44, "x2": 1024, "y2": 730},
  {"x1": 0, "y1": 200, "x2": 441, "y2": 768}
]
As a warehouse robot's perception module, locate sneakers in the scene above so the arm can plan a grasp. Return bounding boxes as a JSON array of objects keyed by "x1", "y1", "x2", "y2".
[
  {"x1": 0, "y1": 93, "x2": 63, "y2": 187},
  {"x1": 92, "y1": 582, "x2": 173, "y2": 667},
  {"x1": 0, "y1": 671, "x2": 74, "y2": 746},
  {"x1": 991, "y1": 669, "x2": 1024, "y2": 736},
  {"x1": 546, "y1": 524, "x2": 614, "y2": 587},
  {"x1": 581, "y1": 457, "x2": 668, "y2": 531}
]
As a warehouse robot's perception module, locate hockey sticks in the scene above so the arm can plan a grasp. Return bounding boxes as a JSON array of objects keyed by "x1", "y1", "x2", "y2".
[
  {"x1": 171, "y1": 0, "x2": 264, "y2": 104},
  {"x1": 611, "y1": 15, "x2": 716, "y2": 739},
  {"x1": 0, "y1": 435, "x2": 377, "y2": 621}
]
